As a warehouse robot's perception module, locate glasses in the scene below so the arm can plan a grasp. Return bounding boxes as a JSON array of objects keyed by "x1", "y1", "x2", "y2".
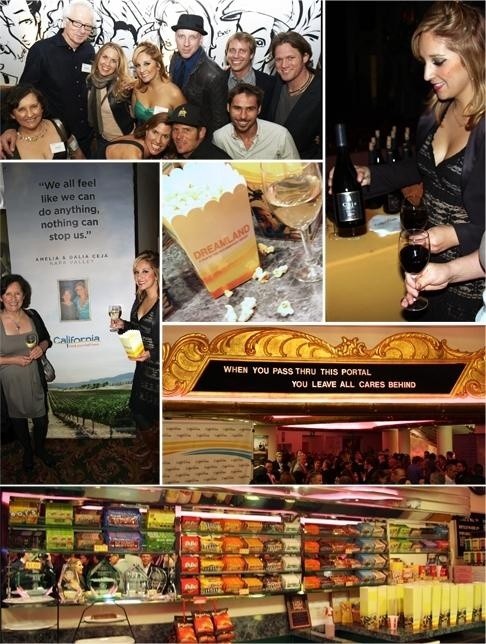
[{"x1": 65, "y1": 15, "x2": 94, "y2": 32}]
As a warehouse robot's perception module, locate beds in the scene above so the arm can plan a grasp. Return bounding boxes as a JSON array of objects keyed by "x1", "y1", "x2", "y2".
[{"x1": 326, "y1": 203, "x2": 416, "y2": 321}]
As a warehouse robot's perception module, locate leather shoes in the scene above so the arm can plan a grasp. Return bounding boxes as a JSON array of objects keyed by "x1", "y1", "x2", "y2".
[
  {"x1": 138, "y1": 455, "x2": 158, "y2": 471},
  {"x1": 134, "y1": 444, "x2": 156, "y2": 459}
]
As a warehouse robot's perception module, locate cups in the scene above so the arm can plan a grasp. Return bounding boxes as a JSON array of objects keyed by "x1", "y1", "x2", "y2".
[{"x1": 387, "y1": 614, "x2": 401, "y2": 630}]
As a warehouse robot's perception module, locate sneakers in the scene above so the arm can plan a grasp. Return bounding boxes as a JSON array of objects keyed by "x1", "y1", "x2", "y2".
[
  {"x1": 21, "y1": 446, "x2": 35, "y2": 470},
  {"x1": 33, "y1": 444, "x2": 57, "y2": 468}
]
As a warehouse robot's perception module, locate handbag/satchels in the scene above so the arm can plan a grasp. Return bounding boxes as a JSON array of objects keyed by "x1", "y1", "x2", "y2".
[{"x1": 38, "y1": 353, "x2": 57, "y2": 383}]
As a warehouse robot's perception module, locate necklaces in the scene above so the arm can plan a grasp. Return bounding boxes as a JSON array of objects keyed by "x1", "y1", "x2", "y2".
[
  {"x1": 3, "y1": 308, "x2": 22, "y2": 332},
  {"x1": 287, "y1": 71, "x2": 311, "y2": 97},
  {"x1": 15, "y1": 121, "x2": 48, "y2": 144}
]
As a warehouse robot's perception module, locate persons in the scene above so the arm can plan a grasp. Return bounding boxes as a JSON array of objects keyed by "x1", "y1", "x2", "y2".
[
  {"x1": 0, "y1": 272, "x2": 56, "y2": 475},
  {"x1": 60, "y1": 281, "x2": 78, "y2": 321},
  {"x1": 111, "y1": 20, "x2": 137, "y2": 78},
  {"x1": 328, "y1": 1, "x2": 484, "y2": 322},
  {"x1": 165, "y1": 102, "x2": 232, "y2": 159},
  {"x1": 135, "y1": 0, "x2": 215, "y2": 74},
  {"x1": 167, "y1": 12, "x2": 227, "y2": 105},
  {"x1": 0, "y1": 0, "x2": 136, "y2": 160},
  {"x1": 218, "y1": 0, "x2": 302, "y2": 74},
  {"x1": 400, "y1": 225, "x2": 485, "y2": 323},
  {"x1": 209, "y1": 83, "x2": 301, "y2": 159},
  {"x1": 89, "y1": 112, "x2": 173, "y2": 160},
  {"x1": 107, "y1": 249, "x2": 159, "y2": 483},
  {"x1": 72, "y1": 281, "x2": 89, "y2": 320},
  {"x1": 0, "y1": 80, "x2": 86, "y2": 159},
  {"x1": 0, "y1": 550, "x2": 181, "y2": 609},
  {"x1": 129, "y1": 40, "x2": 188, "y2": 130},
  {"x1": 0, "y1": 0, "x2": 43, "y2": 50},
  {"x1": 250, "y1": 441, "x2": 485, "y2": 485},
  {"x1": 85, "y1": 42, "x2": 135, "y2": 160},
  {"x1": 264, "y1": 30, "x2": 323, "y2": 157},
  {"x1": 204, "y1": 31, "x2": 274, "y2": 141}
]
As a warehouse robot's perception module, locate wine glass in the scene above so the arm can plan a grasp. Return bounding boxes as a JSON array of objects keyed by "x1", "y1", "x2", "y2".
[
  {"x1": 108, "y1": 304, "x2": 121, "y2": 332},
  {"x1": 25, "y1": 333, "x2": 36, "y2": 358},
  {"x1": 397, "y1": 193, "x2": 430, "y2": 237},
  {"x1": 258, "y1": 161, "x2": 324, "y2": 283},
  {"x1": 397, "y1": 228, "x2": 431, "y2": 312}
]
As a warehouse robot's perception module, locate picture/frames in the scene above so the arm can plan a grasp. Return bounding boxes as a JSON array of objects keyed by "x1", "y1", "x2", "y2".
[{"x1": 284, "y1": 594, "x2": 312, "y2": 632}]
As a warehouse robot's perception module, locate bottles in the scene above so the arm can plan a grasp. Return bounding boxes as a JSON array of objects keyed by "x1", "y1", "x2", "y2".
[
  {"x1": 464, "y1": 540, "x2": 486, "y2": 564},
  {"x1": 331, "y1": 121, "x2": 368, "y2": 239},
  {"x1": 403, "y1": 562, "x2": 419, "y2": 582},
  {"x1": 361, "y1": 125, "x2": 419, "y2": 213}
]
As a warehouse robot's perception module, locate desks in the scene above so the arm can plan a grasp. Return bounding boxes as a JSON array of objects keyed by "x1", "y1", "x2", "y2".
[{"x1": 322, "y1": 618, "x2": 485, "y2": 643}]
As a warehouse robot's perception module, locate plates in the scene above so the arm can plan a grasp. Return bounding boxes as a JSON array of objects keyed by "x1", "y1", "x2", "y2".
[
  {"x1": 0, "y1": 619, "x2": 57, "y2": 632},
  {"x1": 1, "y1": 596, "x2": 55, "y2": 605},
  {"x1": 74, "y1": 636, "x2": 136, "y2": 643},
  {"x1": 81, "y1": 613, "x2": 127, "y2": 623}
]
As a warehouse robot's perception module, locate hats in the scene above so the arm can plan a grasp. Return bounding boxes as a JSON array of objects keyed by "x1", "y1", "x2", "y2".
[
  {"x1": 165, "y1": 102, "x2": 207, "y2": 127},
  {"x1": 169, "y1": 12, "x2": 210, "y2": 36}
]
